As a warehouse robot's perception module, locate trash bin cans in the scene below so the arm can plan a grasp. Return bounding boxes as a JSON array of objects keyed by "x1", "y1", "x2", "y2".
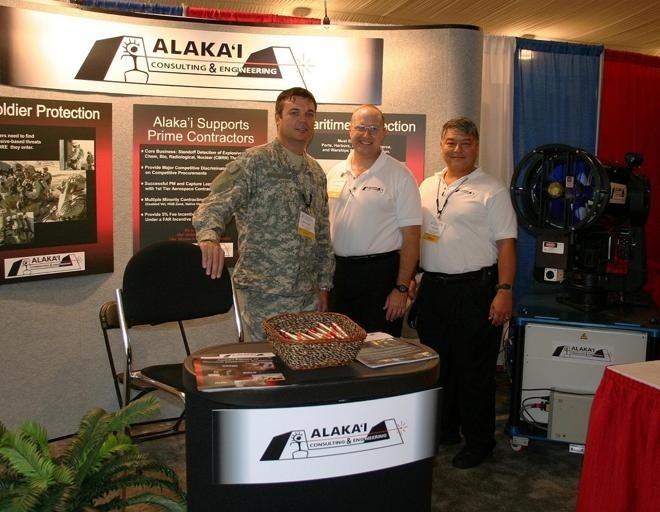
[{"x1": 396, "y1": 285, "x2": 410, "y2": 293}]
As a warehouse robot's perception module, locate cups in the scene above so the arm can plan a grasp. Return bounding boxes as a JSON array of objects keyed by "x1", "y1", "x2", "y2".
[{"x1": 353, "y1": 125, "x2": 383, "y2": 135}]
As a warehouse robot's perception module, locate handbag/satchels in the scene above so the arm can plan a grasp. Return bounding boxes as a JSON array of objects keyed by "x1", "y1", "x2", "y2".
[{"x1": 496, "y1": 284, "x2": 512, "y2": 290}]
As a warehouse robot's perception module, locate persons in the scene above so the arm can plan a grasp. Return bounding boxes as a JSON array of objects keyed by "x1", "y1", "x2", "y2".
[
  {"x1": 0, "y1": 143, "x2": 94, "y2": 225},
  {"x1": 192, "y1": 87, "x2": 336, "y2": 344},
  {"x1": 407, "y1": 117, "x2": 518, "y2": 468},
  {"x1": 325, "y1": 105, "x2": 423, "y2": 337}
]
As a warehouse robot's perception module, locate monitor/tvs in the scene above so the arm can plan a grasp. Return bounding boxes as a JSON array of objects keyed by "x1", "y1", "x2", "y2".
[
  {"x1": 191, "y1": 352, "x2": 290, "y2": 393},
  {"x1": 355, "y1": 331, "x2": 440, "y2": 369}
]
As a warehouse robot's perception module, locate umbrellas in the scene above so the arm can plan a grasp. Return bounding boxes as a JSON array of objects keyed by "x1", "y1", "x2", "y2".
[{"x1": 453, "y1": 445, "x2": 493, "y2": 469}]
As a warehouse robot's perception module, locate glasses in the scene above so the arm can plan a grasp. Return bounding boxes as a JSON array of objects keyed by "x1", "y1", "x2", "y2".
[{"x1": 322, "y1": 0, "x2": 331, "y2": 30}]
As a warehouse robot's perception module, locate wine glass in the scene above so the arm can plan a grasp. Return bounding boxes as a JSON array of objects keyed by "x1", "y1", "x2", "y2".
[{"x1": 504, "y1": 317, "x2": 509, "y2": 322}]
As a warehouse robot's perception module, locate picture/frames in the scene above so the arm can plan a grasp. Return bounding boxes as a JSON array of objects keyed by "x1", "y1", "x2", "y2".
[
  {"x1": 574, "y1": 360, "x2": 660, "y2": 512},
  {"x1": 181, "y1": 338, "x2": 443, "y2": 512}
]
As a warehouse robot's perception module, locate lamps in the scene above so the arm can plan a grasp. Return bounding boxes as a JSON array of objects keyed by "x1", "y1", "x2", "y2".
[
  {"x1": 115, "y1": 239, "x2": 244, "y2": 442},
  {"x1": 99, "y1": 302, "x2": 191, "y2": 435}
]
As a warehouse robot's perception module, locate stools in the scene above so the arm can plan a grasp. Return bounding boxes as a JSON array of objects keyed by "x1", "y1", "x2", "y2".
[{"x1": 425, "y1": 272, "x2": 482, "y2": 279}]
